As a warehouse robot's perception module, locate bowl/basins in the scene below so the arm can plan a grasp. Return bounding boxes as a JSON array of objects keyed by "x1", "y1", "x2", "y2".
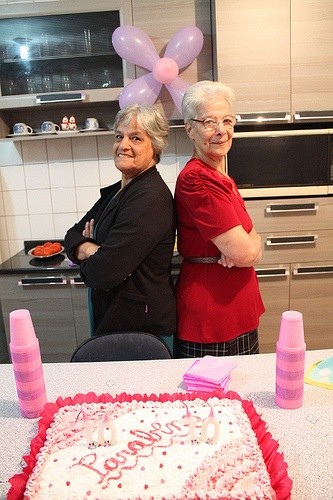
[{"x1": 28, "y1": 246, "x2": 65, "y2": 262}]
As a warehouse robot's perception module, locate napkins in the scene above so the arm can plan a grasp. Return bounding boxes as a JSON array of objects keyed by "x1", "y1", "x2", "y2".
[{"x1": 181, "y1": 353, "x2": 236, "y2": 394}]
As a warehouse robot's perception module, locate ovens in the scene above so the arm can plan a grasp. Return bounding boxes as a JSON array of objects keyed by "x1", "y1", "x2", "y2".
[{"x1": 227, "y1": 121, "x2": 333, "y2": 199}]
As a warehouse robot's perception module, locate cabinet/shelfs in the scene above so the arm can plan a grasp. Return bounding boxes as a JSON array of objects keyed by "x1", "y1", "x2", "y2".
[
  {"x1": 0, "y1": 10, "x2": 133, "y2": 109},
  {"x1": 212, "y1": 0, "x2": 332, "y2": 128},
  {"x1": 238, "y1": 194, "x2": 332, "y2": 354}
]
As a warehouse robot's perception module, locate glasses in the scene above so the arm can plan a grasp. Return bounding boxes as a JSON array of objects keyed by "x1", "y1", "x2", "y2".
[{"x1": 191, "y1": 116, "x2": 237, "y2": 131}]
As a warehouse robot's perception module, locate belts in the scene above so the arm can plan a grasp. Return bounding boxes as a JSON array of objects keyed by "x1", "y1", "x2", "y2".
[{"x1": 184, "y1": 256, "x2": 220, "y2": 263}]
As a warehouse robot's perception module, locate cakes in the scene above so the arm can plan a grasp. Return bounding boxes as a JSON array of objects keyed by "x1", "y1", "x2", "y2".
[{"x1": 4, "y1": 390, "x2": 293, "y2": 500}]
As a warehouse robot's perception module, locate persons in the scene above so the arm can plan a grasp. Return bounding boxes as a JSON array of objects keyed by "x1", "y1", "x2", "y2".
[
  {"x1": 174, "y1": 81, "x2": 266, "y2": 358},
  {"x1": 64, "y1": 103, "x2": 174, "y2": 359}
]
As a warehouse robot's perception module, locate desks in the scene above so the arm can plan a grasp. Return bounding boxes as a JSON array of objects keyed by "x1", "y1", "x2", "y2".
[{"x1": 0, "y1": 348, "x2": 333, "y2": 500}]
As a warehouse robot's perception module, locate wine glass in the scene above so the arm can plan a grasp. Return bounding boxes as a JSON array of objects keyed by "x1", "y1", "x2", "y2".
[{"x1": 55, "y1": 30, "x2": 73, "y2": 55}]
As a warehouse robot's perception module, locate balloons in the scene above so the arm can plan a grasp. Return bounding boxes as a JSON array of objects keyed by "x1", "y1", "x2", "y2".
[
  {"x1": 112, "y1": 24, "x2": 160, "y2": 71},
  {"x1": 119, "y1": 72, "x2": 164, "y2": 109},
  {"x1": 164, "y1": 77, "x2": 193, "y2": 115},
  {"x1": 164, "y1": 26, "x2": 203, "y2": 70},
  {"x1": 153, "y1": 57, "x2": 179, "y2": 83}
]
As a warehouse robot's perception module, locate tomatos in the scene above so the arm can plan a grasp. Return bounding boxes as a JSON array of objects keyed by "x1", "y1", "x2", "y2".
[{"x1": 34, "y1": 242, "x2": 61, "y2": 256}]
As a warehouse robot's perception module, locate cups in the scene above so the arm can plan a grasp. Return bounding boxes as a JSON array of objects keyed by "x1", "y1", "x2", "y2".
[
  {"x1": 85, "y1": 118, "x2": 99, "y2": 129},
  {"x1": 80, "y1": 73, "x2": 91, "y2": 88},
  {"x1": 275, "y1": 310, "x2": 306, "y2": 409},
  {"x1": 26, "y1": 76, "x2": 35, "y2": 92},
  {"x1": 42, "y1": 73, "x2": 53, "y2": 90},
  {"x1": 13, "y1": 123, "x2": 33, "y2": 134},
  {"x1": 4, "y1": 80, "x2": 17, "y2": 95},
  {"x1": 41, "y1": 121, "x2": 60, "y2": 133},
  {"x1": 9, "y1": 309, "x2": 48, "y2": 419},
  {"x1": 39, "y1": 33, "x2": 49, "y2": 57},
  {"x1": 60, "y1": 73, "x2": 72, "y2": 90},
  {"x1": 102, "y1": 72, "x2": 112, "y2": 88},
  {"x1": 80, "y1": 28, "x2": 95, "y2": 53}
]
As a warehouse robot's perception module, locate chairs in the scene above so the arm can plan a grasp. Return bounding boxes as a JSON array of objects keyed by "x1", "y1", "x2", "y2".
[{"x1": 71, "y1": 332, "x2": 171, "y2": 362}]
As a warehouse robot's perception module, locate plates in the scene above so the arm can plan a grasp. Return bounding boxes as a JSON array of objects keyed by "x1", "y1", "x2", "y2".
[
  {"x1": 80, "y1": 128, "x2": 106, "y2": 133},
  {"x1": 7, "y1": 133, "x2": 35, "y2": 137},
  {"x1": 36, "y1": 131, "x2": 59, "y2": 136}
]
